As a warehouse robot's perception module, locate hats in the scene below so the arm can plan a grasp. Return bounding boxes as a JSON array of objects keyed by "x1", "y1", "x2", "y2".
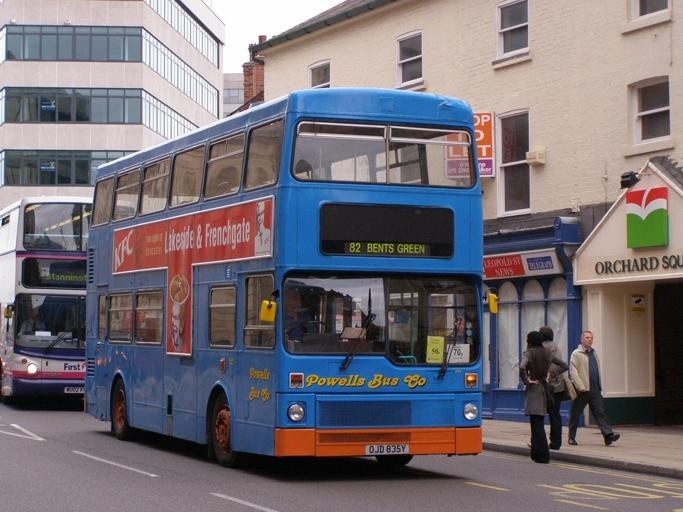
[{"x1": 526, "y1": 330, "x2": 545, "y2": 344}]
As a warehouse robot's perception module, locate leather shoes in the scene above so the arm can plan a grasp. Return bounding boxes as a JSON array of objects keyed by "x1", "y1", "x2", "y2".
[
  {"x1": 603, "y1": 432, "x2": 621, "y2": 446},
  {"x1": 568, "y1": 437, "x2": 577, "y2": 446}
]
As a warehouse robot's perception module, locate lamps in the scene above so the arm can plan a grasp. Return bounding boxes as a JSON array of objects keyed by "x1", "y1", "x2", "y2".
[{"x1": 620, "y1": 172, "x2": 640, "y2": 190}]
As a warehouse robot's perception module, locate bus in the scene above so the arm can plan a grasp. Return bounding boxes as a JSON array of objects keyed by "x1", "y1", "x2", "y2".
[
  {"x1": 0, "y1": 196, "x2": 134, "y2": 403},
  {"x1": 85, "y1": 88, "x2": 500, "y2": 468}
]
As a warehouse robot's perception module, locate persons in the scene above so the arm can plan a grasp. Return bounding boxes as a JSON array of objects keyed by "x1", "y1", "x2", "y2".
[
  {"x1": 518, "y1": 330, "x2": 568, "y2": 464},
  {"x1": 254, "y1": 201, "x2": 270, "y2": 256},
  {"x1": 170, "y1": 302, "x2": 186, "y2": 351},
  {"x1": 537, "y1": 325, "x2": 563, "y2": 449},
  {"x1": 568, "y1": 331, "x2": 620, "y2": 447},
  {"x1": 16, "y1": 306, "x2": 46, "y2": 336}
]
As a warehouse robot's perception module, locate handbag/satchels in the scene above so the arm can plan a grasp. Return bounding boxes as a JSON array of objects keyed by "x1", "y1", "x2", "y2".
[
  {"x1": 543, "y1": 380, "x2": 559, "y2": 415},
  {"x1": 560, "y1": 371, "x2": 578, "y2": 403}
]
